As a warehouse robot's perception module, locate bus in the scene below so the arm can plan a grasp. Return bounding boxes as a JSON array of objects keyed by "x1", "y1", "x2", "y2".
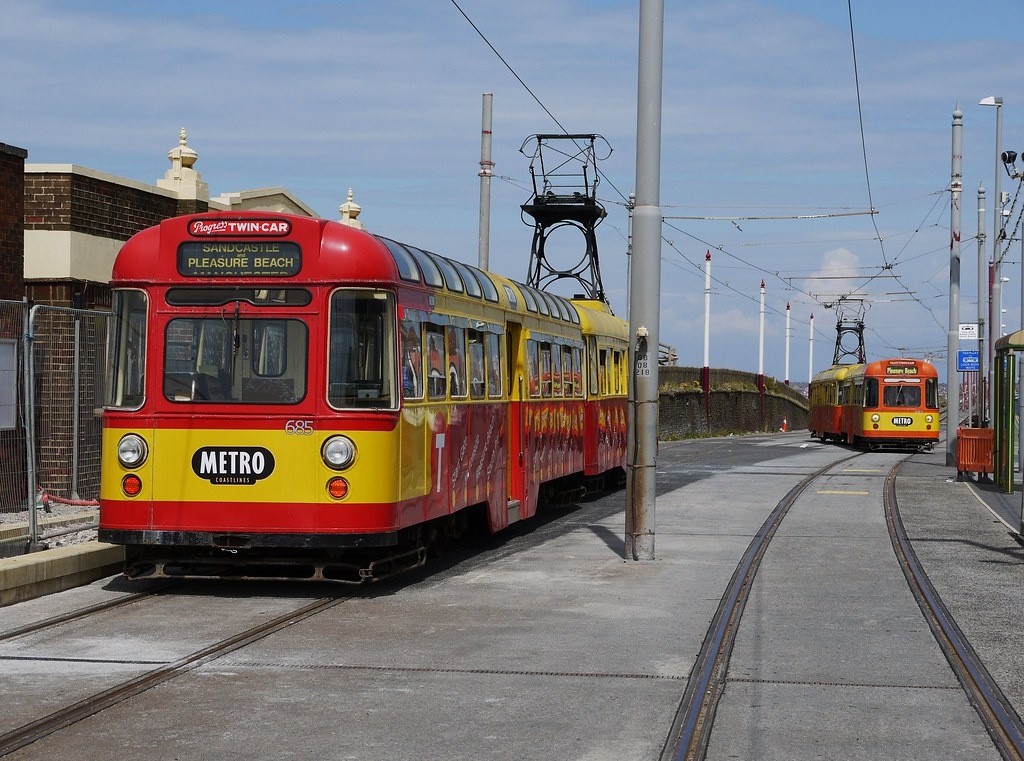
[
  {"x1": 96, "y1": 132, "x2": 680, "y2": 596},
  {"x1": 808, "y1": 312, "x2": 941, "y2": 454}
]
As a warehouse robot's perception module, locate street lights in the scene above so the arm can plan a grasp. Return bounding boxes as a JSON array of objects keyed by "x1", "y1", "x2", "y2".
[{"x1": 980, "y1": 95, "x2": 1004, "y2": 422}]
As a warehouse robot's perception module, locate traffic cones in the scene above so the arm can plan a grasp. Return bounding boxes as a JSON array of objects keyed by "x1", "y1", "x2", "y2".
[{"x1": 780, "y1": 415, "x2": 790, "y2": 432}]
